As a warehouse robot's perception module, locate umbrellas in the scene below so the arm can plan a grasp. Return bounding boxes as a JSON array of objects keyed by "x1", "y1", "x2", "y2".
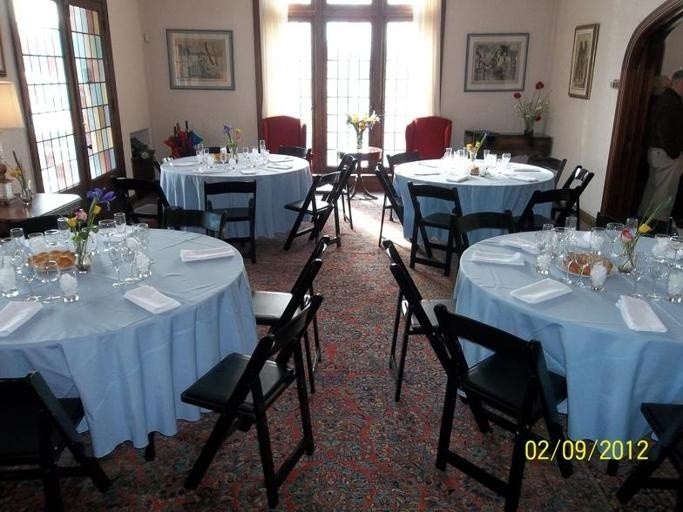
[{"x1": 163, "y1": 121, "x2": 203, "y2": 158}]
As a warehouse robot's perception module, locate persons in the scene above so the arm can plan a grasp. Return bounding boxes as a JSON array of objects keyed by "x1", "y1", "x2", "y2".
[{"x1": 634, "y1": 70, "x2": 683, "y2": 237}]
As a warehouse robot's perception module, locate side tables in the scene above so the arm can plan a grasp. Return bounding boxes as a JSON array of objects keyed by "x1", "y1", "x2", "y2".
[{"x1": 337, "y1": 146, "x2": 382, "y2": 199}]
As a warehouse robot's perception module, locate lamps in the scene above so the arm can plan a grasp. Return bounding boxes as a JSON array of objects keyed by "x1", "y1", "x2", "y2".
[{"x1": 0, "y1": 80, "x2": 25, "y2": 207}]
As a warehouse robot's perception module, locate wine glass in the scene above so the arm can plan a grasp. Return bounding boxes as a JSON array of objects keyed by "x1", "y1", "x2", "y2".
[
  {"x1": 441, "y1": 147, "x2": 511, "y2": 177},
  {"x1": 0, "y1": 213, "x2": 152, "y2": 304},
  {"x1": 536, "y1": 216, "x2": 683, "y2": 304},
  {"x1": 195, "y1": 139, "x2": 269, "y2": 171}
]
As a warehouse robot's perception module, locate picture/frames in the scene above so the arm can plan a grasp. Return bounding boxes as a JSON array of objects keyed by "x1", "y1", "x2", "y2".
[
  {"x1": 464, "y1": 33, "x2": 529, "y2": 91},
  {"x1": 166, "y1": 29, "x2": 234, "y2": 90},
  {"x1": 566, "y1": 24, "x2": 599, "y2": 100}
]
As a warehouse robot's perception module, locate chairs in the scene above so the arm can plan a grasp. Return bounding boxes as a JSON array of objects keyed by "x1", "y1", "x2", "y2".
[
  {"x1": 110, "y1": 143, "x2": 357, "y2": 251},
  {"x1": 406, "y1": 117, "x2": 452, "y2": 160},
  {"x1": 374, "y1": 149, "x2": 594, "y2": 275},
  {"x1": 262, "y1": 116, "x2": 307, "y2": 157}
]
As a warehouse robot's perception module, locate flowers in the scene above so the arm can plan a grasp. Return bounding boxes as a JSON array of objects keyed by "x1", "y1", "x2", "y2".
[
  {"x1": 1, "y1": 148, "x2": 31, "y2": 191},
  {"x1": 620, "y1": 195, "x2": 672, "y2": 267},
  {"x1": 66, "y1": 186, "x2": 116, "y2": 266},
  {"x1": 345, "y1": 110, "x2": 382, "y2": 131},
  {"x1": 223, "y1": 124, "x2": 240, "y2": 146},
  {"x1": 511, "y1": 81, "x2": 554, "y2": 120}
]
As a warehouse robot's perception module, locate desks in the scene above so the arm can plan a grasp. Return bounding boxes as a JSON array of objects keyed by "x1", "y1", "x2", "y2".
[
  {"x1": 0, "y1": 194, "x2": 82, "y2": 239},
  {"x1": 464, "y1": 130, "x2": 551, "y2": 164}
]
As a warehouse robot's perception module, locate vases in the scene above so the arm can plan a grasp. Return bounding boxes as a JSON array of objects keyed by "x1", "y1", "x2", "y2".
[
  {"x1": 524, "y1": 118, "x2": 534, "y2": 137},
  {"x1": 355, "y1": 131, "x2": 363, "y2": 150},
  {"x1": 17, "y1": 175, "x2": 33, "y2": 206}
]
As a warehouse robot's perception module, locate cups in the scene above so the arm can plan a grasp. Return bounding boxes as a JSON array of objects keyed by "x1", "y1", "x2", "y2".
[{"x1": 163, "y1": 157, "x2": 174, "y2": 170}]
